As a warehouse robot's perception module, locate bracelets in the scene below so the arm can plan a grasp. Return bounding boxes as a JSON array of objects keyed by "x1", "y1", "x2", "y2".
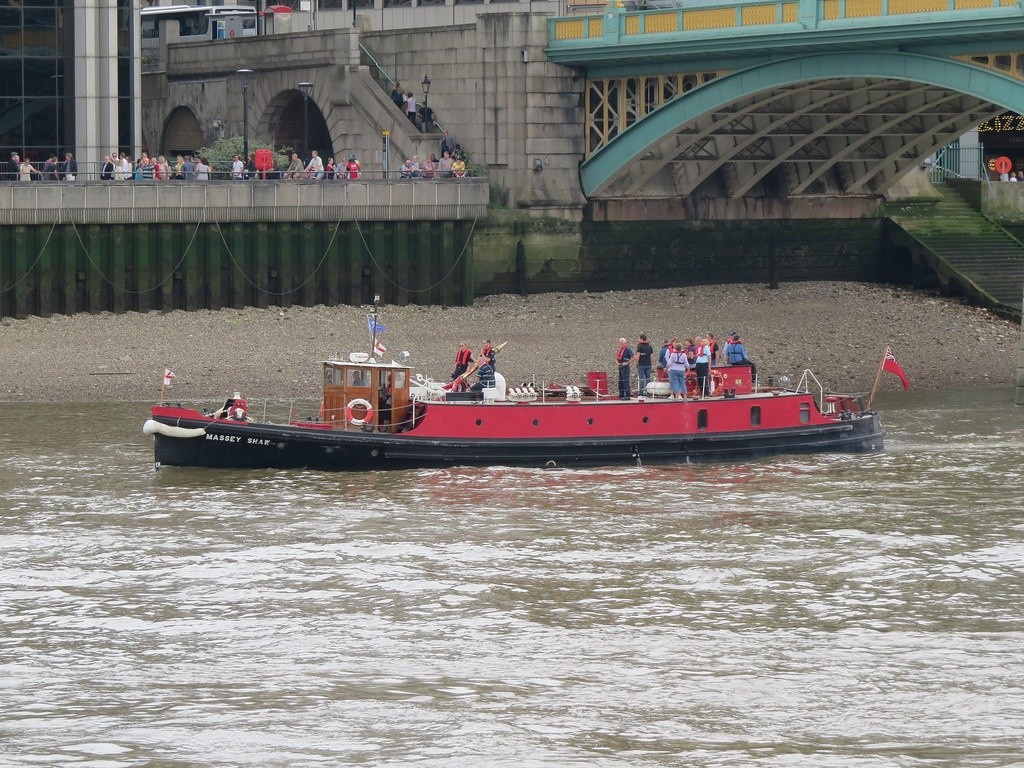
[{"x1": 628, "y1": 360, "x2": 630, "y2": 364}]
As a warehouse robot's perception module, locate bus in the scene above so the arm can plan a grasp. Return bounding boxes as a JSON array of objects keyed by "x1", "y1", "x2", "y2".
[{"x1": 140, "y1": 4, "x2": 258, "y2": 54}]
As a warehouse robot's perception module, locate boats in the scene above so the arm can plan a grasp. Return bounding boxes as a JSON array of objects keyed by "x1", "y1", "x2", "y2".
[{"x1": 143, "y1": 352, "x2": 885, "y2": 470}]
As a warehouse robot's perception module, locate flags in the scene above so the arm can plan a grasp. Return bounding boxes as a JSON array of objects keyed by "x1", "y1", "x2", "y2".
[
  {"x1": 165, "y1": 368, "x2": 176, "y2": 386},
  {"x1": 368, "y1": 317, "x2": 387, "y2": 357},
  {"x1": 882, "y1": 345, "x2": 910, "y2": 391}
]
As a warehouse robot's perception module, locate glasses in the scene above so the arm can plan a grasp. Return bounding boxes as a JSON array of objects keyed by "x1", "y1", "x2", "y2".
[{"x1": 459, "y1": 344, "x2": 466, "y2": 347}]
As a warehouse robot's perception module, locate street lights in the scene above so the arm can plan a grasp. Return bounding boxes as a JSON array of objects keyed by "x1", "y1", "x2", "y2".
[
  {"x1": 237, "y1": 69, "x2": 254, "y2": 178},
  {"x1": 297, "y1": 82, "x2": 314, "y2": 172},
  {"x1": 420, "y1": 73, "x2": 432, "y2": 134}
]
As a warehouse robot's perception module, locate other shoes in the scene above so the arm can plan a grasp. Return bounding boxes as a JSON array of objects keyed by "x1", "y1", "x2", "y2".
[
  {"x1": 678, "y1": 395, "x2": 682, "y2": 400},
  {"x1": 621, "y1": 397, "x2": 630, "y2": 400},
  {"x1": 752, "y1": 380, "x2": 755, "y2": 383},
  {"x1": 668, "y1": 395, "x2": 674, "y2": 400}
]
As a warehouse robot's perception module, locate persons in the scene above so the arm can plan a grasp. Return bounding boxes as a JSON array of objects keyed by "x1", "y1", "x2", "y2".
[
  {"x1": 6, "y1": 151, "x2": 78, "y2": 180},
  {"x1": 352, "y1": 371, "x2": 364, "y2": 386},
  {"x1": 615, "y1": 332, "x2": 757, "y2": 400},
  {"x1": 379, "y1": 374, "x2": 392, "y2": 432},
  {"x1": 100, "y1": 153, "x2": 212, "y2": 180},
  {"x1": 451, "y1": 339, "x2": 496, "y2": 398},
  {"x1": 391, "y1": 82, "x2": 470, "y2": 178},
  {"x1": 1000, "y1": 170, "x2": 1024, "y2": 181},
  {"x1": 230, "y1": 150, "x2": 362, "y2": 181}
]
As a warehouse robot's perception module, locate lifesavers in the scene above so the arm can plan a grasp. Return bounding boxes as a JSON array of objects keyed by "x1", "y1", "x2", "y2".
[
  {"x1": 708, "y1": 370, "x2": 724, "y2": 395},
  {"x1": 685, "y1": 372, "x2": 700, "y2": 395},
  {"x1": 995, "y1": 157, "x2": 1012, "y2": 173},
  {"x1": 346, "y1": 399, "x2": 373, "y2": 426}
]
